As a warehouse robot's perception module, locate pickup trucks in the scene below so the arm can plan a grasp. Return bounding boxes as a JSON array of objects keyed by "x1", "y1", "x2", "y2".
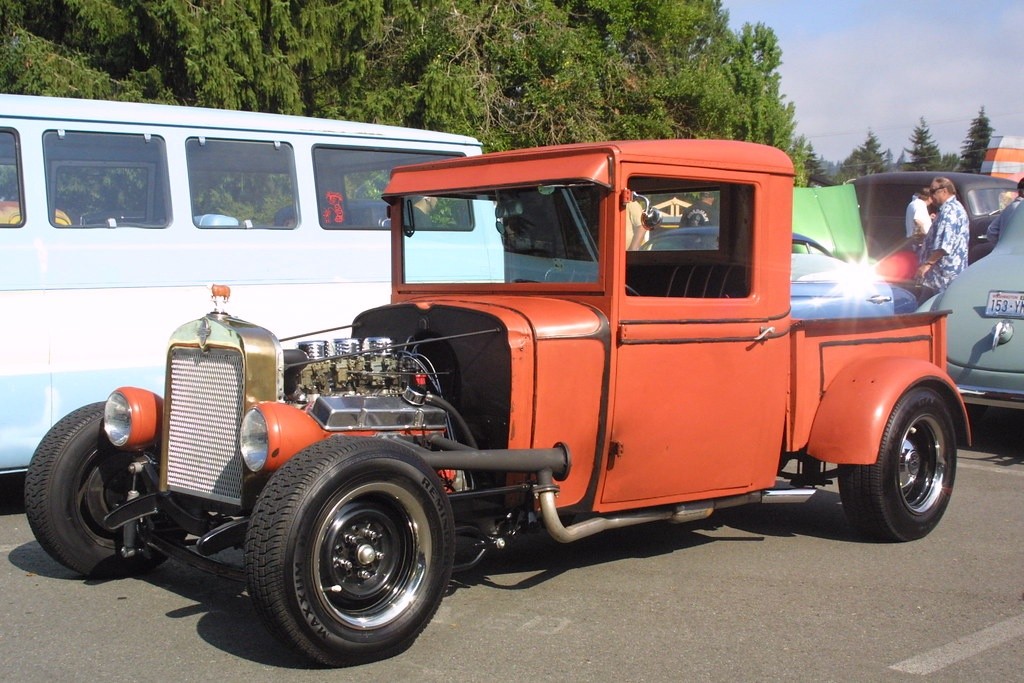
[{"x1": 20, "y1": 137, "x2": 973, "y2": 670}]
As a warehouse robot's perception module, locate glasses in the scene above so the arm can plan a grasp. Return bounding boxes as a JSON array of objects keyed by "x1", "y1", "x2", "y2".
[{"x1": 929, "y1": 186, "x2": 943, "y2": 195}]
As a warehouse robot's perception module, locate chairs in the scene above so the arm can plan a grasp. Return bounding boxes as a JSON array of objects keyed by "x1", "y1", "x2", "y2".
[{"x1": 628, "y1": 263, "x2": 744, "y2": 296}]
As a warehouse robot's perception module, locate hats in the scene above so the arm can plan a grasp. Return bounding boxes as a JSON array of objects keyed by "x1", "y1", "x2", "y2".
[{"x1": 699, "y1": 190, "x2": 714, "y2": 198}]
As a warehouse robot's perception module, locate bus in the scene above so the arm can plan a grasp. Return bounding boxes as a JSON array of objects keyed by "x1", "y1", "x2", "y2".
[{"x1": 0, "y1": 91, "x2": 508, "y2": 491}]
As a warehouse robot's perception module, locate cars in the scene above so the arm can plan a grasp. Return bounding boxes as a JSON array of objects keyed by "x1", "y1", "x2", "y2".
[{"x1": 631, "y1": 168, "x2": 1024, "y2": 420}]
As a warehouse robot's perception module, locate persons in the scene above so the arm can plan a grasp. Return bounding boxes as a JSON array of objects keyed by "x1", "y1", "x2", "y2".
[
  {"x1": 987, "y1": 178, "x2": 1024, "y2": 248},
  {"x1": 413, "y1": 196, "x2": 437, "y2": 229},
  {"x1": 626, "y1": 200, "x2": 647, "y2": 251},
  {"x1": 906, "y1": 176, "x2": 970, "y2": 305},
  {"x1": 680, "y1": 192, "x2": 717, "y2": 228}
]
